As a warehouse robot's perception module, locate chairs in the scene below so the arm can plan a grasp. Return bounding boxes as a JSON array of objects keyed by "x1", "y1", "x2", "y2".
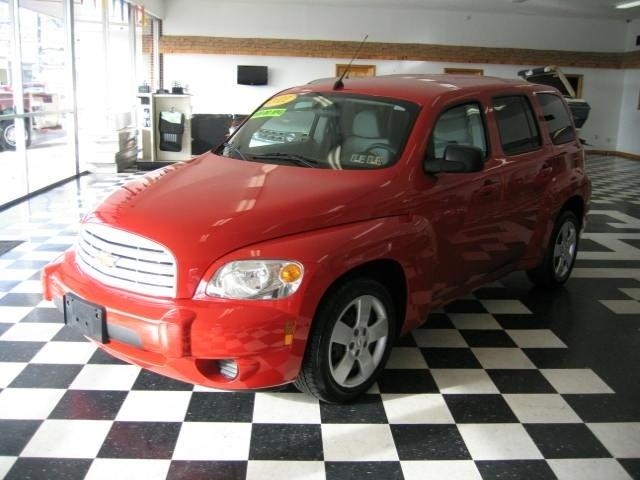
[
  {"x1": 337, "y1": 109, "x2": 397, "y2": 167},
  {"x1": 432, "y1": 110, "x2": 473, "y2": 160}
]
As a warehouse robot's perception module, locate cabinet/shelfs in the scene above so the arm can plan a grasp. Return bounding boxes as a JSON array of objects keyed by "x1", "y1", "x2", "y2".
[{"x1": 136, "y1": 92, "x2": 192, "y2": 162}]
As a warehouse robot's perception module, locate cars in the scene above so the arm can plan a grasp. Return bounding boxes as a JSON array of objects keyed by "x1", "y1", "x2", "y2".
[{"x1": 1, "y1": 86, "x2": 65, "y2": 149}]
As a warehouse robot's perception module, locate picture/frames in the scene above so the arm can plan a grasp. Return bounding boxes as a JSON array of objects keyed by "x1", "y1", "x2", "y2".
[
  {"x1": 336, "y1": 64, "x2": 376, "y2": 77},
  {"x1": 445, "y1": 68, "x2": 483, "y2": 76},
  {"x1": 563, "y1": 74, "x2": 584, "y2": 99}
]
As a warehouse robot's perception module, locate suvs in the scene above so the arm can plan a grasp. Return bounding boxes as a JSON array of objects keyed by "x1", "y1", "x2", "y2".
[{"x1": 40, "y1": 72, "x2": 592, "y2": 403}]
